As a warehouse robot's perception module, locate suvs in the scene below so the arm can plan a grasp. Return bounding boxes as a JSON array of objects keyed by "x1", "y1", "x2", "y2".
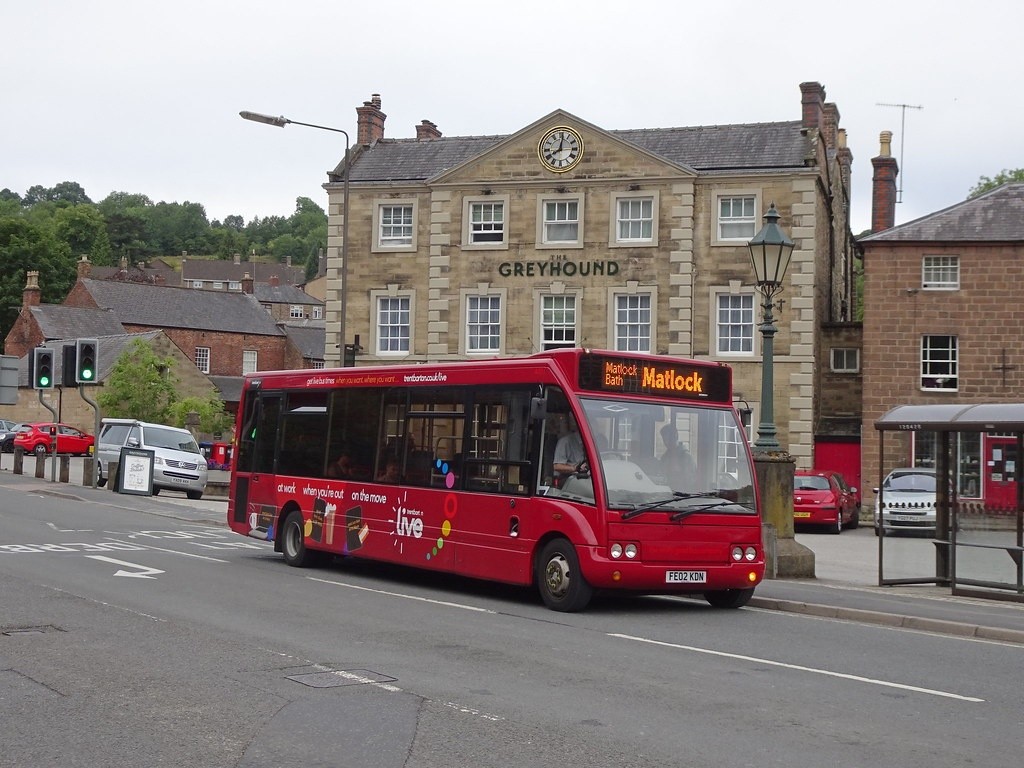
[{"x1": 97, "y1": 418, "x2": 209, "y2": 500}]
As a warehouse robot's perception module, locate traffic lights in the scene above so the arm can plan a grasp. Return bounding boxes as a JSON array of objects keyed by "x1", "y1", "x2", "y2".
[
  {"x1": 32, "y1": 347, "x2": 55, "y2": 390},
  {"x1": 76, "y1": 339, "x2": 97, "y2": 384}
]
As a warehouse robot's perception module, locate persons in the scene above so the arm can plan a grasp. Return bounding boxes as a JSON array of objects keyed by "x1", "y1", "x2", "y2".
[
  {"x1": 328, "y1": 454, "x2": 414, "y2": 486},
  {"x1": 553, "y1": 417, "x2": 608, "y2": 489},
  {"x1": 660, "y1": 424, "x2": 698, "y2": 479}
]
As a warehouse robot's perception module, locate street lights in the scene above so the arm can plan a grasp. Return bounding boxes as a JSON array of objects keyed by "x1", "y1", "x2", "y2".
[
  {"x1": 744, "y1": 201, "x2": 797, "y2": 452},
  {"x1": 238, "y1": 110, "x2": 350, "y2": 369}
]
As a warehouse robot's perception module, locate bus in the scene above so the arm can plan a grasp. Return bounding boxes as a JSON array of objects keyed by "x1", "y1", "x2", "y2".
[{"x1": 227, "y1": 346, "x2": 766, "y2": 614}]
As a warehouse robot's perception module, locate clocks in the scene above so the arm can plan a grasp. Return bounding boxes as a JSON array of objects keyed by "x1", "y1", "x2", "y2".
[{"x1": 537, "y1": 126, "x2": 584, "y2": 173}]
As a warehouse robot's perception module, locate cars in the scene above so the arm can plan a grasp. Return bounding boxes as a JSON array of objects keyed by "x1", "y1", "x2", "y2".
[
  {"x1": 0, "y1": 419, "x2": 27, "y2": 453},
  {"x1": 14, "y1": 421, "x2": 94, "y2": 457},
  {"x1": 794, "y1": 469, "x2": 859, "y2": 534},
  {"x1": 873, "y1": 467, "x2": 960, "y2": 538}
]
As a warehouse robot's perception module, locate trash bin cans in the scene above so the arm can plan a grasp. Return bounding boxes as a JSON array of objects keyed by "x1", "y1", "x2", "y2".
[
  {"x1": 199, "y1": 441, "x2": 213, "y2": 460},
  {"x1": 213, "y1": 442, "x2": 226, "y2": 464},
  {"x1": 227, "y1": 445, "x2": 232, "y2": 465}
]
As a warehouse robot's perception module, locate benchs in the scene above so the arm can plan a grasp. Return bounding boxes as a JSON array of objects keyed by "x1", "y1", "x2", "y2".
[{"x1": 931, "y1": 540, "x2": 1024, "y2": 565}]
